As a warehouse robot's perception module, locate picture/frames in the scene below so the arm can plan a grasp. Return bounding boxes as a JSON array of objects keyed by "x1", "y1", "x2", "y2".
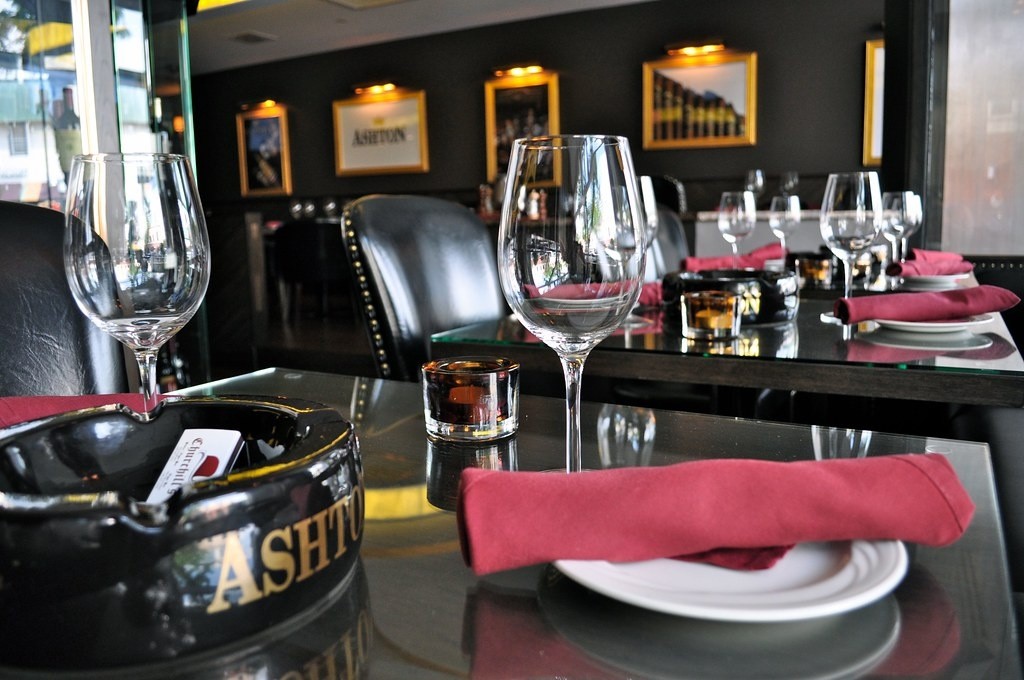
[
  {"x1": 642, "y1": 51, "x2": 757, "y2": 150},
  {"x1": 236, "y1": 108, "x2": 292, "y2": 195},
  {"x1": 333, "y1": 90, "x2": 428, "y2": 176},
  {"x1": 484, "y1": 72, "x2": 561, "y2": 190},
  {"x1": 863, "y1": 40, "x2": 884, "y2": 166}
]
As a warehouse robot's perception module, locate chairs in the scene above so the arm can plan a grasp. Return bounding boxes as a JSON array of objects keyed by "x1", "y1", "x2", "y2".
[
  {"x1": 642, "y1": 206, "x2": 691, "y2": 280},
  {"x1": 340, "y1": 194, "x2": 507, "y2": 380},
  {"x1": 0, "y1": 197, "x2": 129, "y2": 401}
]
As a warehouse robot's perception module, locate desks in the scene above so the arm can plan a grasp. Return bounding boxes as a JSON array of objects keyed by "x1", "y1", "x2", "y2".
[{"x1": 161, "y1": 367, "x2": 1024, "y2": 680}]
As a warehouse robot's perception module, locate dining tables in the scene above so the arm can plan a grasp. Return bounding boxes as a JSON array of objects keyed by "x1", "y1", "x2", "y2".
[{"x1": 429, "y1": 262, "x2": 1024, "y2": 428}]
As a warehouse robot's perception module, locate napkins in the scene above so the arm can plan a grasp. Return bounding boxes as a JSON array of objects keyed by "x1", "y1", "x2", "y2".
[
  {"x1": 681, "y1": 254, "x2": 764, "y2": 274},
  {"x1": 833, "y1": 286, "x2": 1020, "y2": 325},
  {"x1": 455, "y1": 452, "x2": 977, "y2": 573},
  {"x1": 461, "y1": 558, "x2": 957, "y2": 680},
  {"x1": 0, "y1": 394, "x2": 174, "y2": 434},
  {"x1": 886, "y1": 261, "x2": 973, "y2": 277},
  {"x1": 748, "y1": 243, "x2": 787, "y2": 260},
  {"x1": 511, "y1": 310, "x2": 666, "y2": 342},
  {"x1": 843, "y1": 333, "x2": 1017, "y2": 363},
  {"x1": 905, "y1": 248, "x2": 963, "y2": 262}
]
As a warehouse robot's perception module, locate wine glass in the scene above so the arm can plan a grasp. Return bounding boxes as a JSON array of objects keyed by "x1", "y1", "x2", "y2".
[
  {"x1": 63, "y1": 150, "x2": 212, "y2": 415},
  {"x1": 289, "y1": 198, "x2": 337, "y2": 224},
  {"x1": 718, "y1": 168, "x2": 923, "y2": 328},
  {"x1": 596, "y1": 174, "x2": 659, "y2": 329},
  {"x1": 497, "y1": 133, "x2": 647, "y2": 476}
]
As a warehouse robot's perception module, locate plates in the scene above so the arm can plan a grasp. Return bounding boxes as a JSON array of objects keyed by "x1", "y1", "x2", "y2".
[
  {"x1": 552, "y1": 537, "x2": 908, "y2": 624},
  {"x1": 853, "y1": 329, "x2": 993, "y2": 352},
  {"x1": 895, "y1": 282, "x2": 968, "y2": 292},
  {"x1": 906, "y1": 274, "x2": 971, "y2": 282},
  {"x1": 872, "y1": 314, "x2": 994, "y2": 333},
  {"x1": 536, "y1": 570, "x2": 900, "y2": 679}
]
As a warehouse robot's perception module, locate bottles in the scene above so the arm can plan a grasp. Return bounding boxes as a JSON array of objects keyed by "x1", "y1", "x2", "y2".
[
  {"x1": 478, "y1": 185, "x2": 548, "y2": 221},
  {"x1": 159, "y1": 339, "x2": 190, "y2": 393}
]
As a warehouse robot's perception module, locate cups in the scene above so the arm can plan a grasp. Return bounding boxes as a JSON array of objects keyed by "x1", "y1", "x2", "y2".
[
  {"x1": 421, "y1": 355, "x2": 521, "y2": 443},
  {"x1": 596, "y1": 403, "x2": 655, "y2": 469},
  {"x1": 811, "y1": 424, "x2": 872, "y2": 462},
  {"x1": 680, "y1": 291, "x2": 745, "y2": 340},
  {"x1": 425, "y1": 435, "x2": 519, "y2": 514}
]
